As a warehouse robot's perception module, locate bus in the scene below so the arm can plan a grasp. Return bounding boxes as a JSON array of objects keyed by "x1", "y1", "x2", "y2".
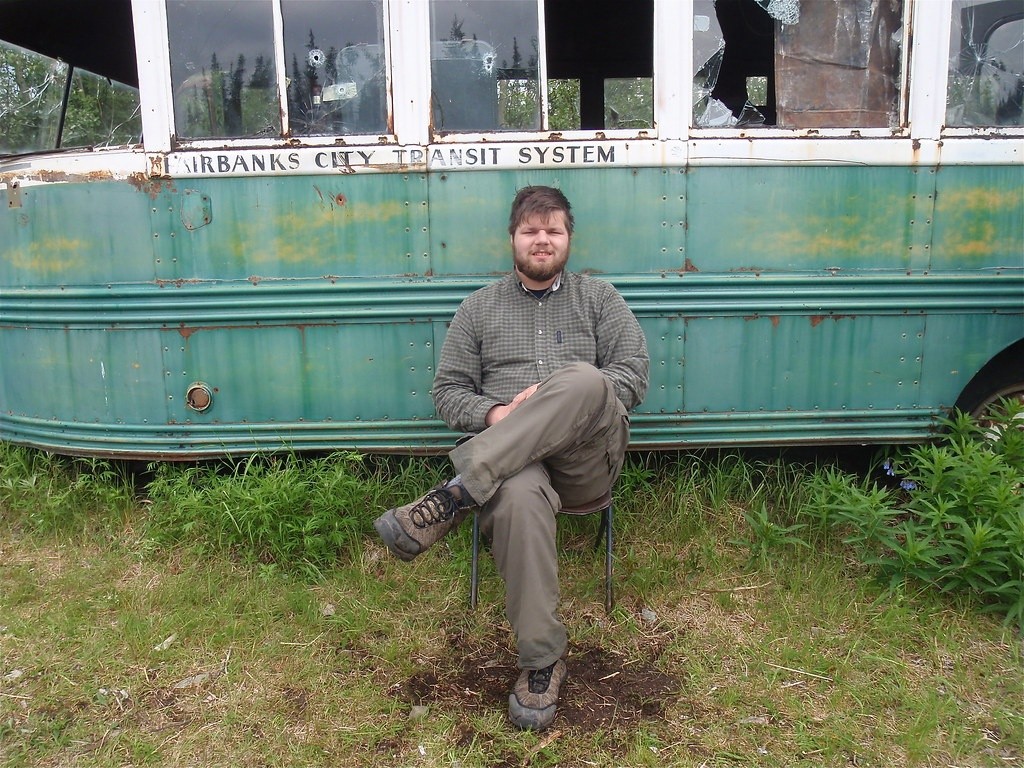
[{"x1": 1, "y1": 2, "x2": 1024, "y2": 472}]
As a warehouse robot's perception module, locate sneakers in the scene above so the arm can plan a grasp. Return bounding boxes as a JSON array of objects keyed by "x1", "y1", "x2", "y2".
[
  {"x1": 373, "y1": 479, "x2": 478, "y2": 563},
  {"x1": 508, "y1": 647, "x2": 568, "y2": 730}
]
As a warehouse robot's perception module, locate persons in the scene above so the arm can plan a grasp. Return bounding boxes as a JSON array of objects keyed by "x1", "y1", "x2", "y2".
[{"x1": 374, "y1": 186, "x2": 651, "y2": 730}]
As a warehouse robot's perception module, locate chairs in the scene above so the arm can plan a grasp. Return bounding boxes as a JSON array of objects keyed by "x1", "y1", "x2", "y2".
[{"x1": 446, "y1": 321, "x2": 615, "y2": 614}]
